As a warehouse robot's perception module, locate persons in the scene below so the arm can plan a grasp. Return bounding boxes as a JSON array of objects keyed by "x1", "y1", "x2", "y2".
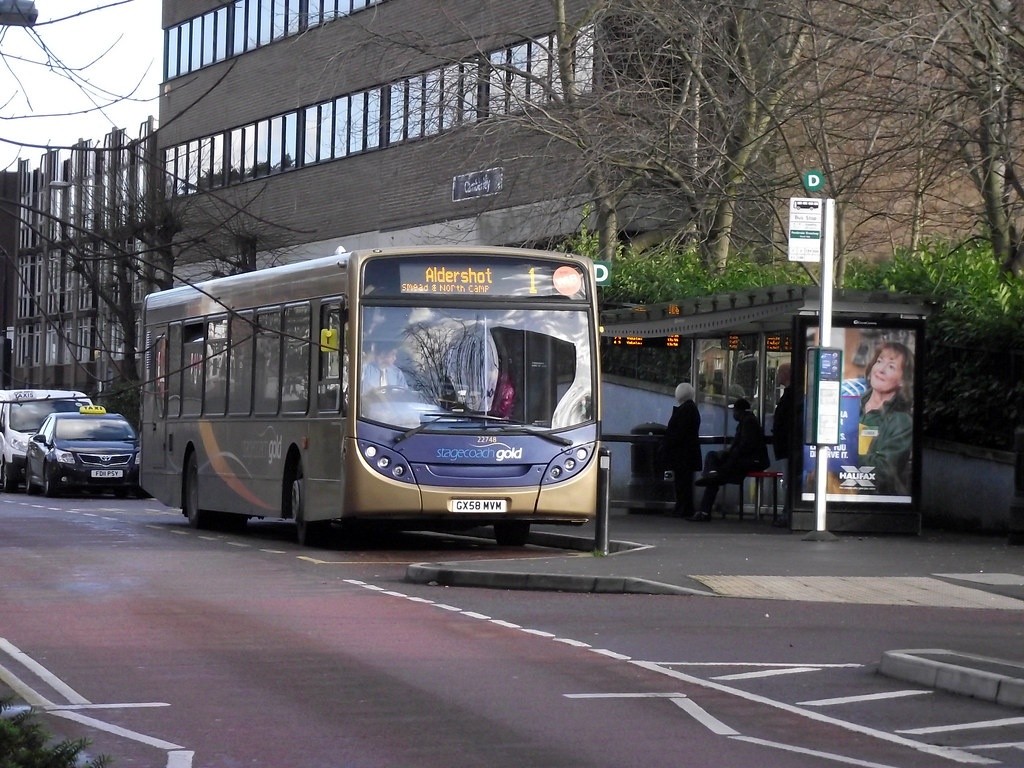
[
  {"x1": 687, "y1": 397, "x2": 770, "y2": 522},
  {"x1": 804, "y1": 340, "x2": 914, "y2": 496},
  {"x1": 362, "y1": 341, "x2": 409, "y2": 396},
  {"x1": 773, "y1": 363, "x2": 792, "y2": 526},
  {"x1": 481, "y1": 362, "x2": 516, "y2": 424},
  {"x1": 663, "y1": 382, "x2": 702, "y2": 518}
]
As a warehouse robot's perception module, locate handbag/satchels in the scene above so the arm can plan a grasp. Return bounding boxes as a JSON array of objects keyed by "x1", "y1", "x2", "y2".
[{"x1": 747, "y1": 449, "x2": 770, "y2": 471}]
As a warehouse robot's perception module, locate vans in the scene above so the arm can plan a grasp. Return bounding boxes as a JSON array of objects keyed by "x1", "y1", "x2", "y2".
[{"x1": 0, "y1": 388, "x2": 95, "y2": 494}]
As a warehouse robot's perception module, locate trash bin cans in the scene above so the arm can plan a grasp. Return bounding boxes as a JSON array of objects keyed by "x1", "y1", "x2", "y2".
[{"x1": 628, "y1": 421, "x2": 670, "y2": 515}]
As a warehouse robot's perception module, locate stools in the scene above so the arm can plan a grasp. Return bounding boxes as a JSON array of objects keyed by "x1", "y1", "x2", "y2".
[{"x1": 736, "y1": 471, "x2": 779, "y2": 524}]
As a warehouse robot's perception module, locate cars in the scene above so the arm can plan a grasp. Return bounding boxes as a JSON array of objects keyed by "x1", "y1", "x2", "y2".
[{"x1": 25, "y1": 404, "x2": 140, "y2": 498}]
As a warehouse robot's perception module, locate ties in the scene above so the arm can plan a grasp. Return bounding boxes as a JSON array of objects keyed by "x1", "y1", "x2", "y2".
[{"x1": 379, "y1": 368, "x2": 388, "y2": 393}]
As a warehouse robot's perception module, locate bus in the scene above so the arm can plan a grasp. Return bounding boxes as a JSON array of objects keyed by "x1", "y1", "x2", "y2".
[{"x1": 137, "y1": 244, "x2": 606, "y2": 550}]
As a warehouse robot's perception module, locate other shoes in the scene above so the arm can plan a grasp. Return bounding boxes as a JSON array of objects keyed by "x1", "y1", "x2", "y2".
[
  {"x1": 694, "y1": 471, "x2": 720, "y2": 487},
  {"x1": 685, "y1": 511, "x2": 712, "y2": 521}
]
